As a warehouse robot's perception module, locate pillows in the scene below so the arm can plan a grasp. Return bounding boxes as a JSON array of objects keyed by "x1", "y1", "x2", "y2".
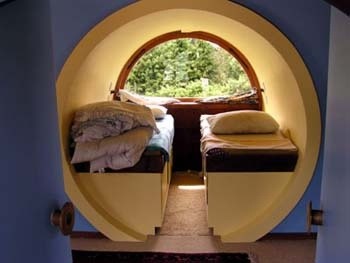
[
  {"x1": 75, "y1": 101, "x2": 160, "y2": 135},
  {"x1": 207, "y1": 110, "x2": 280, "y2": 134},
  {"x1": 146, "y1": 105, "x2": 168, "y2": 118}
]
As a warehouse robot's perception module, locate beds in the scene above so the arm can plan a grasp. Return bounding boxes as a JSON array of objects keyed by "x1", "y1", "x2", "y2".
[
  {"x1": 66, "y1": 115, "x2": 174, "y2": 236},
  {"x1": 201, "y1": 115, "x2": 299, "y2": 236}
]
